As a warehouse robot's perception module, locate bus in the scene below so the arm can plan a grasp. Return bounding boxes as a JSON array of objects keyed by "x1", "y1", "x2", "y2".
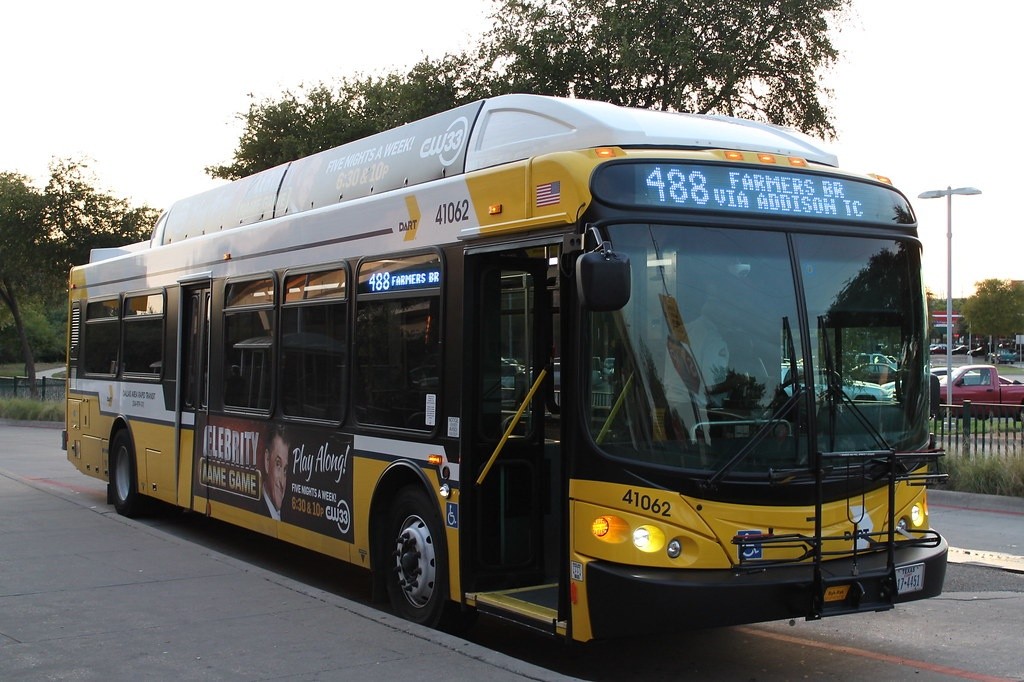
[{"x1": 60, "y1": 90, "x2": 949, "y2": 645}]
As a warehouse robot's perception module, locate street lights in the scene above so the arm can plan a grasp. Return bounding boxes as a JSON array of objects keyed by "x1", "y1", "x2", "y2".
[{"x1": 917, "y1": 186, "x2": 982, "y2": 428}]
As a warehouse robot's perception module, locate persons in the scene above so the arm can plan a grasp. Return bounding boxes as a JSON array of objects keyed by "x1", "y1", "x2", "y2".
[
  {"x1": 665, "y1": 308, "x2": 729, "y2": 445},
  {"x1": 259, "y1": 425, "x2": 290, "y2": 520}
]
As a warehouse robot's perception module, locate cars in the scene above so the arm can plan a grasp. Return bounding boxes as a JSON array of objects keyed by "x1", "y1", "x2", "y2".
[
  {"x1": 779, "y1": 349, "x2": 903, "y2": 405},
  {"x1": 930, "y1": 341, "x2": 1020, "y2": 366}
]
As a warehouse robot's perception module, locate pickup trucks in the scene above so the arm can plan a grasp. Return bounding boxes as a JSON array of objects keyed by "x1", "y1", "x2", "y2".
[{"x1": 936, "y1": 364, "x2": 1023, "y2": 423}]
